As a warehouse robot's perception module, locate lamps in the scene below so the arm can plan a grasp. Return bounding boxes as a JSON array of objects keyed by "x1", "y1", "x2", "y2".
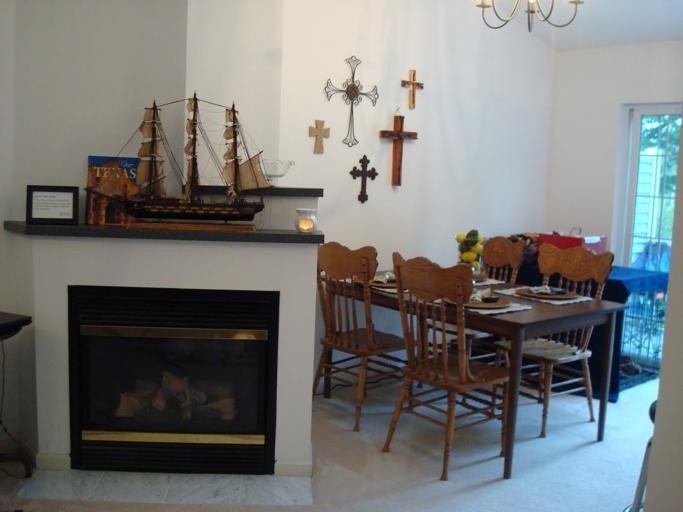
[{"x1": 476, "y1": 1, "x2": 586, "y2": 35}]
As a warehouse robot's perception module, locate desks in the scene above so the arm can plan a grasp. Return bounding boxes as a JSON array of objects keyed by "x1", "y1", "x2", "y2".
[{"x1": 324, "y1": 262, "x2": 626, "y2": 478}]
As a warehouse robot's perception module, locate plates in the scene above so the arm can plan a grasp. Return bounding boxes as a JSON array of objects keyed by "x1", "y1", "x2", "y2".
[
  {"x1": 441, "y1": 295, "x2": 510, "y2": 308},
  {"x1": 515, "y1": 287, "x2": 579, "y2": 300}
]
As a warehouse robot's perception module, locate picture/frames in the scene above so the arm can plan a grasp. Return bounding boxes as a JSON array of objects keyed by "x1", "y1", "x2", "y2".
[{"x1": 26, "y1": 184, "x2": 80, "y2": 224}]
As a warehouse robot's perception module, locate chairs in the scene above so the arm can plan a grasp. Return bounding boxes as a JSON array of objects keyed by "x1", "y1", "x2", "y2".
[
  {"x1": 319, "y1": 240, "x2": 417, "y2": 435},
  {"x1": 487, "y1": 242, "x2": 612, "y2": 438},
  {"x1": 417, "y1": 237, "x2": 529, "y2": 411},
  {"x1": 380, "y1": 250, "x2": 522, "y2": 478}
]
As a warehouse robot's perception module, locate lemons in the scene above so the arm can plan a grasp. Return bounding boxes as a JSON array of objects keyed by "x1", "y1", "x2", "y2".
[{"x1": 455, "y1": 230, "x2": 484, "y2": 270}]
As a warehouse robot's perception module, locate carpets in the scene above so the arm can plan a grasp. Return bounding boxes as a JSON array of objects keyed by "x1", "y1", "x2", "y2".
[{"x1": 19, "y1": 469, "x2": 312, "y2": 506}]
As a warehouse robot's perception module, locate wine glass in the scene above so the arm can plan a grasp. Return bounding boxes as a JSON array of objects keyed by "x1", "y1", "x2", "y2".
[{"x1": 262, "y1": 159, "x2": 294, "y2": 187}]
